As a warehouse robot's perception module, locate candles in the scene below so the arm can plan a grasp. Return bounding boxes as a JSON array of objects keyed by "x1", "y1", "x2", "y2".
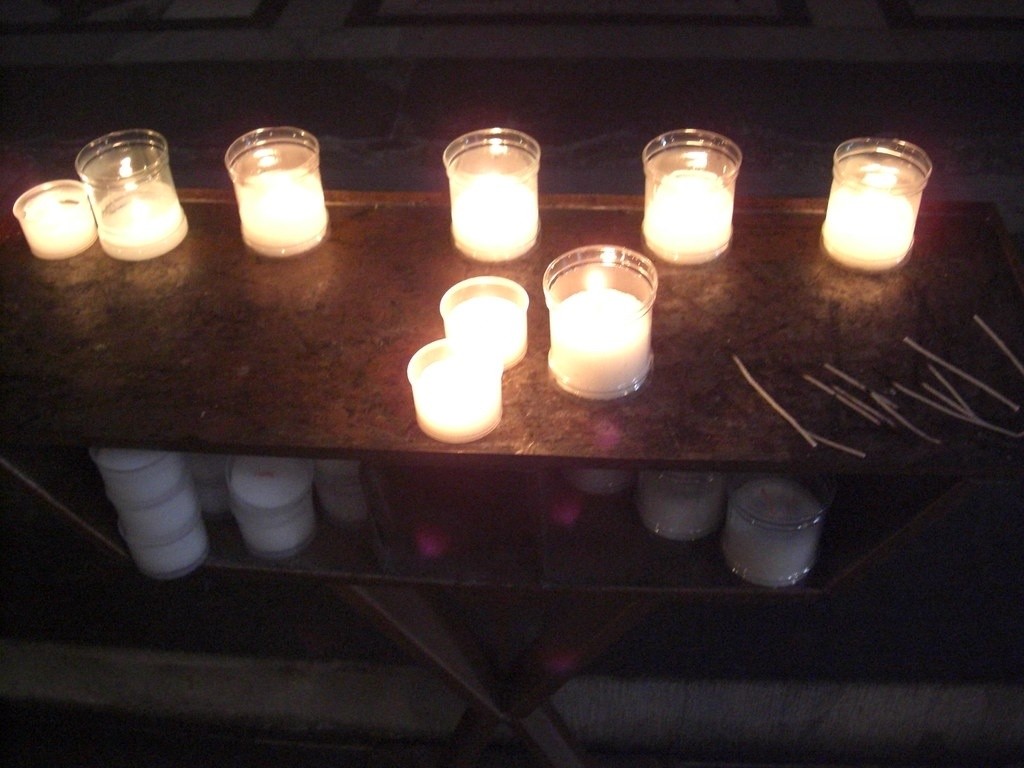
[
  {"x1": 548, "y1": 266, "x2": 654, "y2": 400},
  {"x1": 94, "y1": 448, "x2": 827, "y2": 586},
  {"x1": 450, "y1": 172, "x2": 539, "y2": 263},
  {"x1": 23, "y1": 191, "x2": 99, "y2": 260},
  {"x1": 820, "y1": 163, "x2": 917, "y2": 273},
  {"x1": 95, "y1": 157, "x2": 189, "y2": 262},
  {"x1": 240, "y1": 168, "x2": 329, "y2": 259},
  {"x1": 643, "y1": 169, "x2": 735, "y2": 266},
  {"x1": 444, "y1": 295, "x2": 529, "y2": 371},
  {"x1": 414, "y1": 358, "x2": 504, "y2": 443}
]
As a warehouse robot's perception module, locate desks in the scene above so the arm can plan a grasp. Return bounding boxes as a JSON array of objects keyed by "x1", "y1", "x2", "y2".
[{"x1": 0, "y1": 185, "x2": 1024, "y2": 768}]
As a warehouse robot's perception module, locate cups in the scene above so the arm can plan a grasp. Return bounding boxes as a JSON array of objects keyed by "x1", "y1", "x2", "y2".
[
  {"x1": 542, "y1": 244, "x2": 659, "y2": 401},
  {"x1": 439, "y1": 276, "x2": 529, "y2": 371},
  {"x1": 406, "y1": 338, "x2": 503, "y2": 443},
  {"x1": 75, "y1": 130, "x2": 189, "y2": 261},
  {"x1": 821, "y1": 138, "x2": 932, "y2": 271},
  {"x1": 642, "y1": 128, "x2": 743, "y2": 265},
  {"x1": 225, "y1": 127, "x2": 329, "y2": 257},
  {"x1": 443, "y1": 128, "x2": 541, "y2": 263},
  {"x1": 13, "y1": 179, "x2": 98, "y2": 259}
]
